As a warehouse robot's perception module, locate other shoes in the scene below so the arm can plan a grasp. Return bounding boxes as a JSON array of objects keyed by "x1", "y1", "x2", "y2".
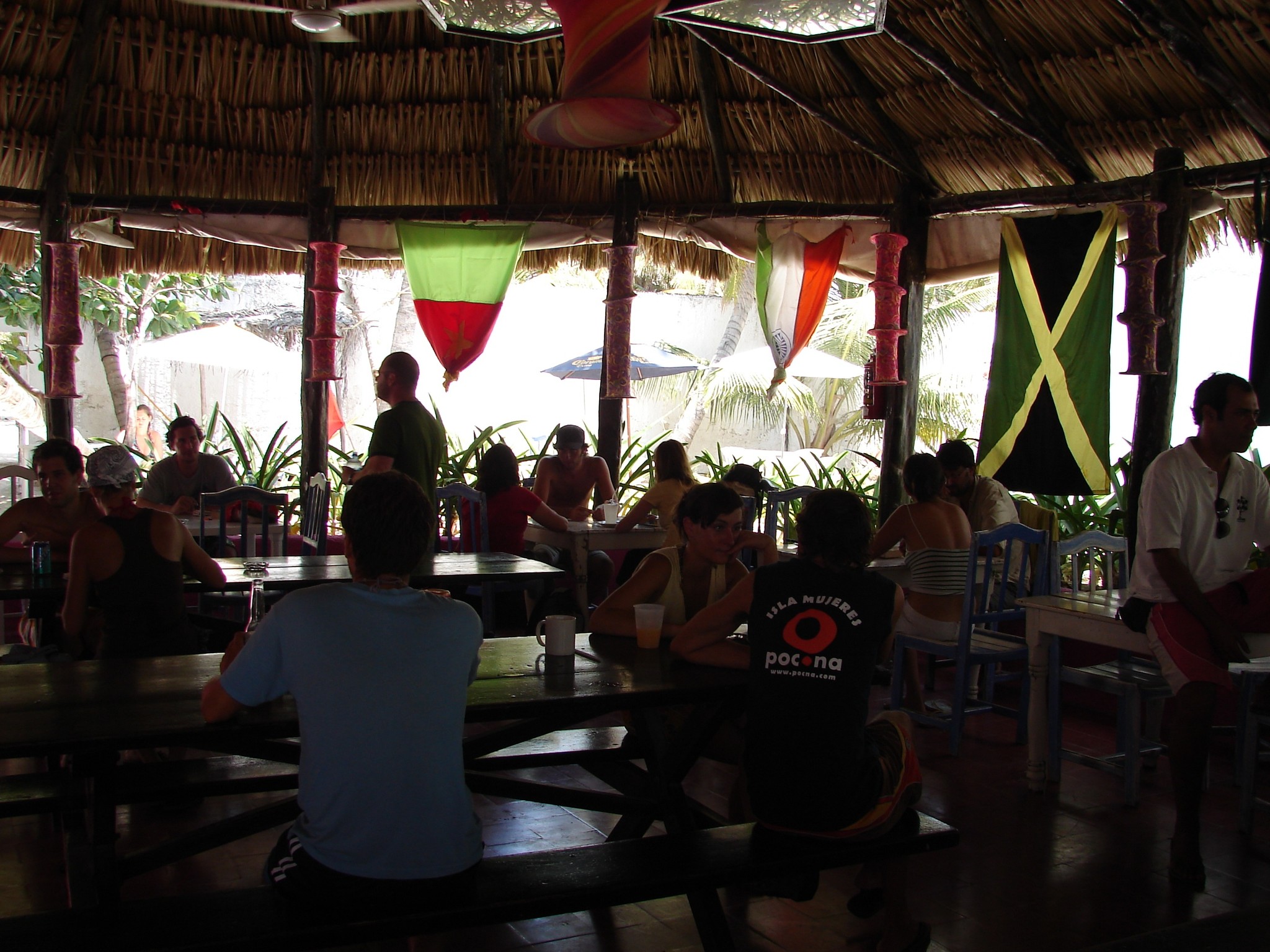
[
  {"x1": 1169, "y1": 838, "x2": 1208, "y2": 891},
  {"x1": 876, "y1": 661, "x2": 894, "y2": 671}
]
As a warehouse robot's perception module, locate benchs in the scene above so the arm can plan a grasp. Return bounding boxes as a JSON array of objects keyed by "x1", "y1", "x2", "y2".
[{"x1": 0, "y1": 727, "x2": 960, "y2": 952}]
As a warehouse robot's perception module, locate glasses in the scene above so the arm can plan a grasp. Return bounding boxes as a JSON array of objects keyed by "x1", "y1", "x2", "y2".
[{"x1": 1214, "y1": 498, "x2": 1232, "y2": 539}]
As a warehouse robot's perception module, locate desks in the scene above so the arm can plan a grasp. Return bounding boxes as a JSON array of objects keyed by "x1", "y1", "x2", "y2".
[
  {"x1": 1015, "y1": 588, "x2": 1270, "y2": 845},
  {"x1": 181, "y1": 552, "x2": 565, "y2": 654},
  {"x1": 777, "y1": 544, "x2": 1005, "y2": 708},
  {"x1": 522, "y1": 516, "x2": 667, "y2": 634},
  {"x1": 176, "y1": 514, "x2": 291, "y2": 557},
  {"x1": 0, "y1": 631, "x2": 748, "y2": 952}
]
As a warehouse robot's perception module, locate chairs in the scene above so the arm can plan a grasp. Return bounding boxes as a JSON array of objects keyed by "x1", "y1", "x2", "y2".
[
  {"x1": 435, "y1": 478, "x2": 1270, "y2": 832},
  {"x1": 1, "y1": 466, "x2": 331, "y2": 556}
]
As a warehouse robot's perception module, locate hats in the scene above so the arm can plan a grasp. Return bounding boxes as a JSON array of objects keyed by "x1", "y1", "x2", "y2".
[
  {"x1": 555, "y1": 425, "x2": 585, "y2": 451},
  {"x1": 87, "y1": 446, "x2": 134, "y2": 488}
]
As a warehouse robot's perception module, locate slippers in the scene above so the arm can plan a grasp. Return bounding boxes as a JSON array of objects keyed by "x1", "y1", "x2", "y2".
[
  {"x1": 909, "y1": 922, "x2": 932, "y2": 952},
  {"x1": 846, "y1": 884, "x2": 888, "y2": 917},
  {"x1": 924, "y1": 698, "x2": 953, "y2": 715}
]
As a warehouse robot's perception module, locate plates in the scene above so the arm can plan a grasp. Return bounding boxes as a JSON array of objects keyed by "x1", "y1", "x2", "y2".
[{"x1": 597, "y1": 521, "x2": 620, "y2": 527}]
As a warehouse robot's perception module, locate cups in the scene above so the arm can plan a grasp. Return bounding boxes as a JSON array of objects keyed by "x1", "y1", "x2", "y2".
[
  {"x1": 536, "y1": 615, "x2": 577, "y2": 655},
  {"x1": 603, "y1": 503, "x2": 619, "y2": 524},
  {"x1": 633, "y1": 603, "x2": 666, "y2": 649}
]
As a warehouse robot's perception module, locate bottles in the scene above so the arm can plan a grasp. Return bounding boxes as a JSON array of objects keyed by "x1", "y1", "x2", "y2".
[{"x1": 243, "y1": 579, "x2": 266, "y2": 645}]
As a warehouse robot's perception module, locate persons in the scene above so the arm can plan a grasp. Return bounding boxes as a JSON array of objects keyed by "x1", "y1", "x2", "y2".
[
  {"x1": 868, "y1": 454, "x2": 973, "y2": 713},
  {"x1": 668, "y1": 488, "x2": 929, "y2": 952},
  {"x1": 1121, "y1": 374, "x2": 1270, "y2": 890},
  {"x1": 203, "y1": 470, "x2": 483, "y2": 952},
  {"x1": 0, "y1": 353, "x2": 768, "y2": 763},
  {"x1": 588, "y1": 482, "x2": 779, "y2": 824},
  {"x1": 936, "y1": 440, "x2": 1031, "y2": 611}
]
